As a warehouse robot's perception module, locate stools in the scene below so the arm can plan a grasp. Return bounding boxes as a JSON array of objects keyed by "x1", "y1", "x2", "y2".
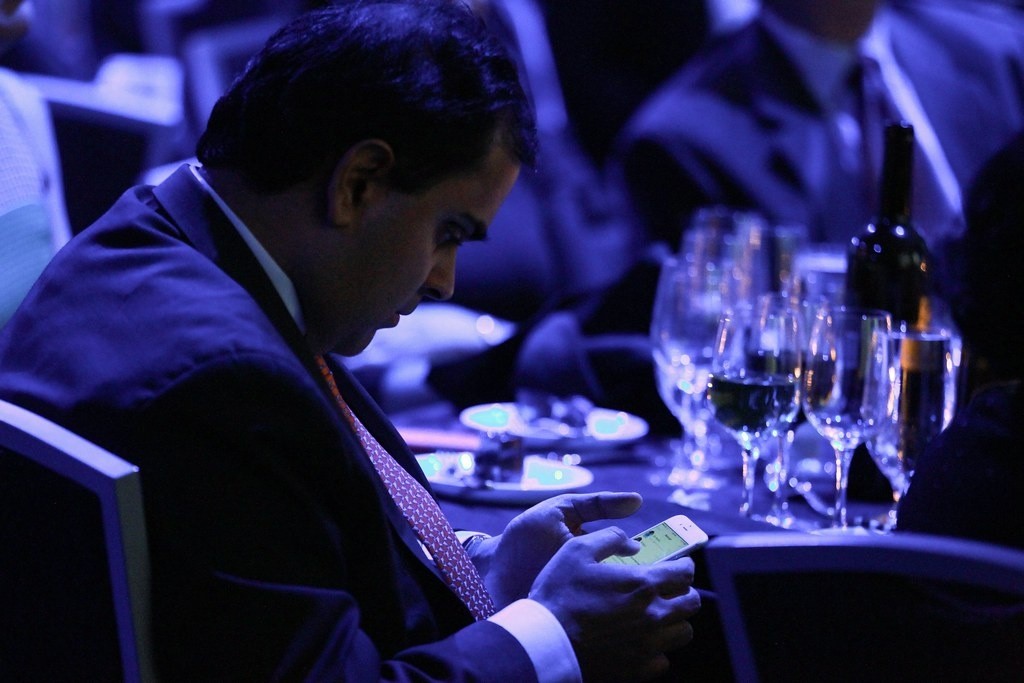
[{"x1": 0, "y1": 396, "x2": 158, "y2": 683}]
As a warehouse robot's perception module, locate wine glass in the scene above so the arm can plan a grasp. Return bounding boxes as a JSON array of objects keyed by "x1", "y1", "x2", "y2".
[
  {"x1": 706, "y1": 288, "x2": 838, "y2": 533},
  {"x1": 645, "y1": 203, "x2": 764, "y2": 493},
  {"x1": 800, "y1": 303, "x2": 894, "y2": 541},
  {"x1": 783, "y1": 249, "x2": 852, "y2": 420},
  {"x1": 861, "y1": 328, "x2": 957, "y2": 537}
]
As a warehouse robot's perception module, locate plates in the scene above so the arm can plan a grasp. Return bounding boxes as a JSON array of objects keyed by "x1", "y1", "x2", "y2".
[
  {"x1": 456, "y1": 397, "x2": 649, "y2": 461},
  {"x1": 410, "y1": 447, "x2": 595, "y2": 505},
  {"x1": 389, "y1": 421, "x2": 484, "y2": 452}
]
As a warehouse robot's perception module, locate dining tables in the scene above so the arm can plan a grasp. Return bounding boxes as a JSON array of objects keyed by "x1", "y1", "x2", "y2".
[{"x1": 388, "y1": 405, "x2": 913, "y2": 683}]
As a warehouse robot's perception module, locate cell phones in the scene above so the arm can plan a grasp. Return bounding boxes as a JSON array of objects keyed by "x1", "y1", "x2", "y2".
[{"x1": 599, "y1": 514, "x2": 708, "y2": 567}]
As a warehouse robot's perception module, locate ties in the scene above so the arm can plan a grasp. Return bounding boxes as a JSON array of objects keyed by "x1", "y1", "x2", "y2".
[
  {"x1": 307, "y1": 333, "x2": 495, "y2": 624},
  {"x1": 843, "y1": 61, "x2": 959, "y2": 268}
]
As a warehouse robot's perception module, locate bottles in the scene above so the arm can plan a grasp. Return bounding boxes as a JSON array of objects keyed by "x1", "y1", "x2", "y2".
[{"x1": 843, "y1": 120, "x2": 935, "y2": 499}]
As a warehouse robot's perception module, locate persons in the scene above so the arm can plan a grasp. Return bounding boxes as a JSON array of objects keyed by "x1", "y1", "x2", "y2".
[
  {"x1": 619, "y1": 0, "x2": 993, "y2": 257},
  {"x1": 0, "y1": 0, "x2": 701, "y2": 683}
]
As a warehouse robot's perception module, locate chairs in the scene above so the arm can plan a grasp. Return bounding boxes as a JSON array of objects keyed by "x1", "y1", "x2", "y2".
[{"x1": 706, "y1": 529, "x2": 1024, "y2": 682}]
